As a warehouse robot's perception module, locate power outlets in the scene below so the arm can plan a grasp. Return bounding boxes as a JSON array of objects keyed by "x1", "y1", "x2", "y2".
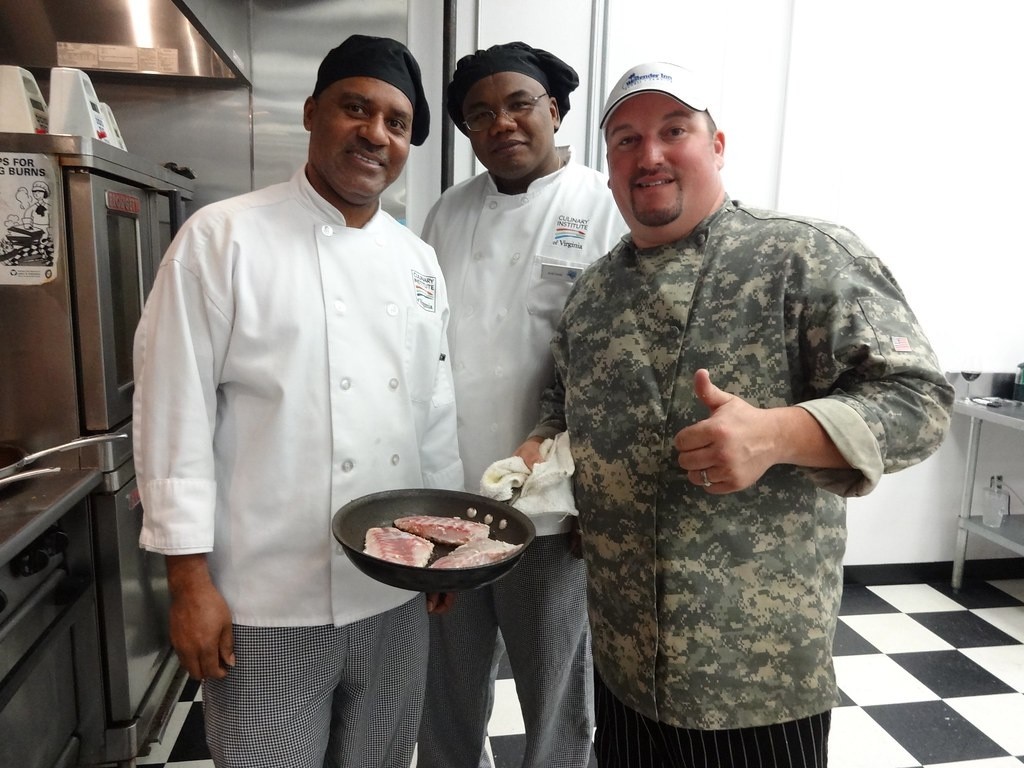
[{"x1": 990, "y1": 475, "x2": 1003, "y2": 497}]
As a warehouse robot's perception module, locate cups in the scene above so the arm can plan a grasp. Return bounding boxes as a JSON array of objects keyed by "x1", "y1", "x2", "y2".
[{"x1": 983, "y1": 487, "x2": 1008, "y2": 528}]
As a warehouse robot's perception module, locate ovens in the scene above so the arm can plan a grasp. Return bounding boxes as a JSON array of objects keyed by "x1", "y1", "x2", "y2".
[{"x1": 0, "y1": 522, "x2": 89, "y2": 768}]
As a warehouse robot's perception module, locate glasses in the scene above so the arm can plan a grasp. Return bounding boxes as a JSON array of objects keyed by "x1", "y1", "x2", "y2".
[{"x1": 463, "y1": 94, "x2": 546, "y2": 132}]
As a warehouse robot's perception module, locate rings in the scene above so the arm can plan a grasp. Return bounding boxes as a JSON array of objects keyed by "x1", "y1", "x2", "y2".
[{"x1": 700, "y1": 470, "x2": 712, "y2": 487}]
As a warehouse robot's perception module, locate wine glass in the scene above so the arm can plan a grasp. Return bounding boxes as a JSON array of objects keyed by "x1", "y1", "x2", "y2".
[{"x1": 958, "y1": 370, "x2": 981, "y2": 404}]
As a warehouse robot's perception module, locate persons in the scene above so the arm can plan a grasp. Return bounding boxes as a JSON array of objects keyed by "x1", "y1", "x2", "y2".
[
  {"x1": 512, "y1": 61, "x2": 955, "y2": 768},
  {"x1": 416, "y1": 43, "x2": 632, "y2": 768},
  {"x1": 132, "y1": 34, "x2": 465, "y2": 768}
]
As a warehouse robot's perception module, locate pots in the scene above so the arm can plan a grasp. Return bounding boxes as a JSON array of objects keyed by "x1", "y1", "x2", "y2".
[
  {"x1": 0, "y1": 434, "x2": 128, "y2": 480},
  {"x1": 332, "y1": 488, "x2": 535, "y2": 593}
]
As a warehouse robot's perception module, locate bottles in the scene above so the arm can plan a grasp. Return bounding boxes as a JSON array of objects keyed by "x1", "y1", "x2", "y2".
[{"x1": 1013, "y1": 362, "x2": 1024, "y2": 403}]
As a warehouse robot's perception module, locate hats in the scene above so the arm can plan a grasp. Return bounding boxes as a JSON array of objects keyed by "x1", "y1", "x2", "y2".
[
  {"x1": 311, "y1": 34, "x2": 430, "y2": 146},
  {"x1": 446, "y1": 41, "x2": 580, "y2": 134},
  {"x1": 598, "y1": 61, "x2": 708, "y2": 129}
]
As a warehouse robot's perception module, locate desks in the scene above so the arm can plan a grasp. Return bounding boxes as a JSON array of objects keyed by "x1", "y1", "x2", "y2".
[{"x1": 951, "y1": 395, "x2": 1024, "y2": 591}]
